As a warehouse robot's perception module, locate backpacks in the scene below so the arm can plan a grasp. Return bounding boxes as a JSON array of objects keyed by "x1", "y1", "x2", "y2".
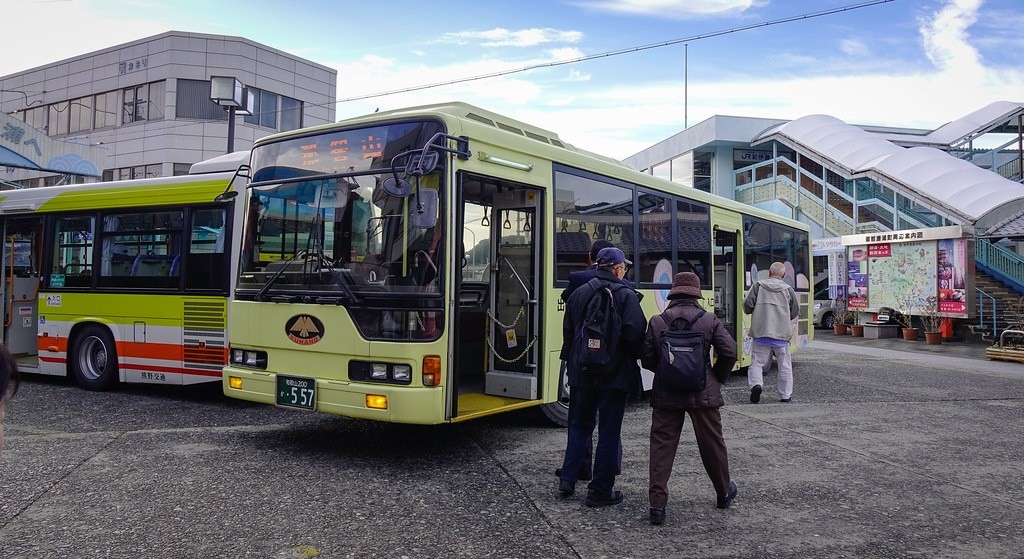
[
  {"x1": 572, "y1": 277, "x2": 639, "y2": 374},
  {"x1": 654, "y1": 309, "x2": 707, "y2": 396}
]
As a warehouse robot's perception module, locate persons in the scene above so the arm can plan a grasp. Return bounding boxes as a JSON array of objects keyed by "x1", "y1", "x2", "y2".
[
  {"x1": 555, "y1": 240, "x2": 636, "y2": 488},
  {"x1": 641, "y1": 272, "x2": 738, "y2": 525},
  {"x1": 559, "y1": 248, "x2": 648, "y2": 506},
  {"x1": 742, "y1": 262, "x2": 799, "y2": 404}
]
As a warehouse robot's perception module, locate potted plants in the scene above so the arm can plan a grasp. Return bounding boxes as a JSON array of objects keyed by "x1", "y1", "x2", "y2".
[
  {"x1": 850, "y1": 308, "x2": 867, "y2": 336},
  {"x1": 892, "y1": 295, "x2": 920, "y2": 340},
  {"x1": 832, "y1": 307, "x2": 851, "y2": 335},
  {"x1": 918, "y1": 296, "x2": 950, "y2": 345}
]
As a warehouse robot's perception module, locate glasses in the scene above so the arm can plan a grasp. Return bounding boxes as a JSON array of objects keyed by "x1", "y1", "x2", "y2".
[{"x1": 615, "y1": 266, "x2": 628, "y2": 272}]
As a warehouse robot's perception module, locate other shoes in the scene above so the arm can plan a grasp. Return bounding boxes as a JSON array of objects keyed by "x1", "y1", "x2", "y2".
[
  {"x1": 716, "y1": 483, "x2": 737, "y2": 507},
  {"x1": 749, "y1": 386, "x2": 762, "y2": 403},
  {"x1": 780, "y1": 396, "x2": 793, "y2": 403},
  {"x1": 584, "y1": 490, "x2": 623, "y2": 506},
  {"x1": 648, "y1": 507, "x2": 667, "y2": 522},
  {"x1": 558, "y1": 479, "x2": 577, "y2": 494}
]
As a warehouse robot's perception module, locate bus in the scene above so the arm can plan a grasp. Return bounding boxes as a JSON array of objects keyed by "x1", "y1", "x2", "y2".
[
  {"x1": 211, "y1": 98, "x2": 816, "y2": 432},
  {"x1": 0, "y1": 147, "x2": 379, "y2": 393}
]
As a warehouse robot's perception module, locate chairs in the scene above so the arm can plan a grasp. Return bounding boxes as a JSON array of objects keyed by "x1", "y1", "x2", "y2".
[{"x1": 111, "y1": 253, "x2": 181, "y2": 276}]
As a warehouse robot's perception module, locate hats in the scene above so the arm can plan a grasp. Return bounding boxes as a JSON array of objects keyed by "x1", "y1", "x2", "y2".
[
  {"x1": 667, "y1": 271, "x2": 705, "y2": 299},
  {"x1": 595, "y1": 247, "x2": 633, "y2": 268}
]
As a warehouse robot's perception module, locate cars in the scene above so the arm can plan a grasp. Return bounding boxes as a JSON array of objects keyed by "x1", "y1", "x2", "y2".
[{"x1": 813, "y1": 287, "x2": 836, "y2": 330}]
